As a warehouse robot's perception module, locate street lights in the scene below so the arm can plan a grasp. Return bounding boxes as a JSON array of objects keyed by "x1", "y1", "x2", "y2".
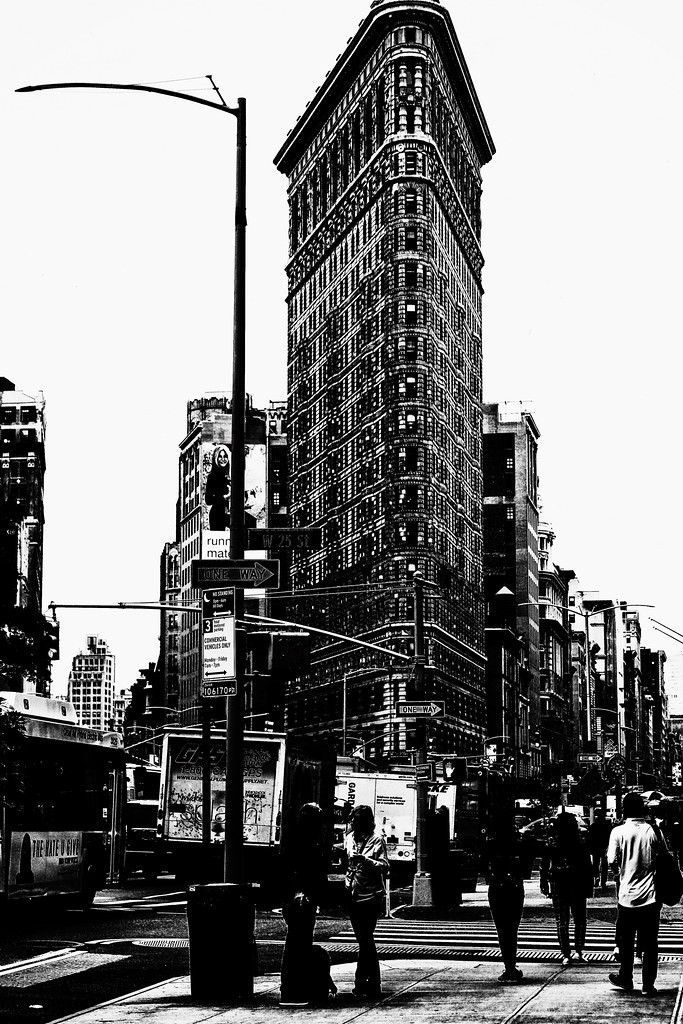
[{"x1": 518, "y1": 602, "x2": 656, "y2": 749}]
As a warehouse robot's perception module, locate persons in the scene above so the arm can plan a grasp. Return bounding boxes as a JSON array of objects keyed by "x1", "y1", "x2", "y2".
[
  {"x1": 606, "y1": 793, "x2": 669, "y2": 993},
  {"x1": 280, "y1": 802, "x2": 327, "y2": 1004},
  {"x1": 469, "y1": 816, "x2": 538, "y2": 981},
  {"x1": 329, "y1": 804, "x2": 389, "y2": 996},
  {"x1": 539, "y1": 813, "x2": 591, "y2": 964},
  {"x1": 585, "y1": 808, "x2": 612, "y2": 890}
]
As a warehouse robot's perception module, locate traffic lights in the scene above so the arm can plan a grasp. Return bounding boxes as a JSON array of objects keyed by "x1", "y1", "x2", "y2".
[{"x1": 43, "y1": 621, "x2": 59, "y2": 660}]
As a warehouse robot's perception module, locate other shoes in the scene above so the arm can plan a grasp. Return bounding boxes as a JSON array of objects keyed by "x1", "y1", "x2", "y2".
[
  {"x1": 498, "y1": 968, "x2": 523, "y2": 980},
  {"x1": 563, "y1": 957, "x2": 572, "y2": 966},
  {"x1": 642, "y1": 985, "x2": 657, "y2": 995},
  {"x1": 609, "y1": 973, "x2": 633, "y2": 990}
]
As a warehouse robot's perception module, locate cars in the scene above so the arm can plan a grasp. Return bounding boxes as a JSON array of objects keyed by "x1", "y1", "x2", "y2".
[{"x1": 513, "y1": 790, "x2": 673, "y2": 845}]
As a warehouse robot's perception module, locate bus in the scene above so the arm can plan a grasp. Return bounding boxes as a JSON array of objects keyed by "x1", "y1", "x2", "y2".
[
  {"x1": 158, "y1": 723, "x2": 330, "y2": 877},
  {"x1": 0, "y1": 690, "x2": 146, "y2": 917}
]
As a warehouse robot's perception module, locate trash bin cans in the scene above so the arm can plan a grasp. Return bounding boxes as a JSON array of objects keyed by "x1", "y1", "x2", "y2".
[{"x1": 188, "y1": 881, "x2": 257, "y2": 1007}]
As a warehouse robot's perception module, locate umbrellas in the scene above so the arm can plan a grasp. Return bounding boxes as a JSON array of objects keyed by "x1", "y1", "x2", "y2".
[{"x1": 640, "y1": 791, "x2": 665, "y2": 800}]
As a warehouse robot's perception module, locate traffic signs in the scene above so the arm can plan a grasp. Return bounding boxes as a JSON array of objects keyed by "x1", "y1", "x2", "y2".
[
  {"x1": 395, "y1": 701, "x2": 445, "y2": 718},
  {"x1": 245, "y1": 527, "x2": 323, "y2": 550},
  {"x1": 190, "y1": 559, "x2": 281, "y2": 590},
  {"x1": 576, "y1": 753, "x2": 604, "y2": 763}
]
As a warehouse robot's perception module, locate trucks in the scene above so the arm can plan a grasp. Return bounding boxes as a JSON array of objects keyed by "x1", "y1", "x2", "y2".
[{"x1": 333, "y1": 755, "x2": 492, "y2": 892}]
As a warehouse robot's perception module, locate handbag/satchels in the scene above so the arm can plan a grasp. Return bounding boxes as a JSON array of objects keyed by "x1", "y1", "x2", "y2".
[{"x1": 646, "y1": 819, "x2": 682, "y2": 906}]
